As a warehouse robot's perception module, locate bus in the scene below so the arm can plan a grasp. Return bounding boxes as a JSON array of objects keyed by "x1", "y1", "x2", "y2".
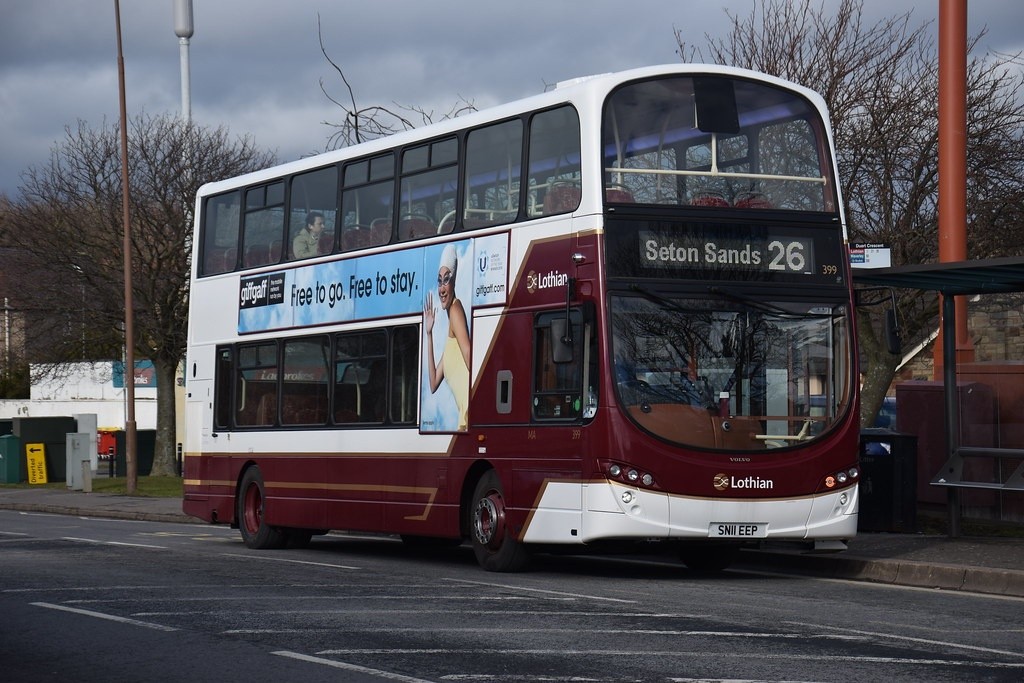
[{"x1": 183, "y1": 64, "x2": 901, "y2": 573}]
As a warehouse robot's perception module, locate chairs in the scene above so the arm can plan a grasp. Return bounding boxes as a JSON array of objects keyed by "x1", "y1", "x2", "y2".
[
  {"x1": 236, "y1": 387, "x2": 359, "y2": 424},
  {"x1": 205, "y1": 176, "x2": 775, "y2": 277}
]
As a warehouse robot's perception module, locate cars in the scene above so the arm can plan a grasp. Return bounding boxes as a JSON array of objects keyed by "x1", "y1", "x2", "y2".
[{"x1": 800, "y1": 395, "x2": 896, "y2": 442}]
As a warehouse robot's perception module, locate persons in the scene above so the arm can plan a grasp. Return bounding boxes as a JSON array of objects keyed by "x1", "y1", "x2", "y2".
[
  {"x1": 292, "y1": 211, "x2": 335, "y2": 259},
  {"x1": 336, "y1": 209, "x2": 357, "y2": 246},
  {"x1": 674, "y1": 354, "x2": 717, "y2": 413},
  {"x1": 857, "y1": 371, "x2": 866, "y2": 392},
  {"x1": 422, "y1": 244, "x2": 472, "y2": 436}
]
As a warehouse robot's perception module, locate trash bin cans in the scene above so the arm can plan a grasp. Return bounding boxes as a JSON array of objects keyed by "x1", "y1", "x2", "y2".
[
  {"x1": 860, "y1": 428, "x2": 919, "y2": 535},
  {"x1": 0, "y1": 433, "x2": 22, "y2": 485}
]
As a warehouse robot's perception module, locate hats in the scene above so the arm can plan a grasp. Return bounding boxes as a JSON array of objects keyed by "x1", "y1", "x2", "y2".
[{"x1": 438, "y1": 243, "x2": 458, "y2": 279}]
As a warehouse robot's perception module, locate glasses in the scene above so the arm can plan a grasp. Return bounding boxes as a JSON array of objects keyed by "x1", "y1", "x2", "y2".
[{"x1": 437, "y1": 277, "x2": 453, "y2": 287}]
